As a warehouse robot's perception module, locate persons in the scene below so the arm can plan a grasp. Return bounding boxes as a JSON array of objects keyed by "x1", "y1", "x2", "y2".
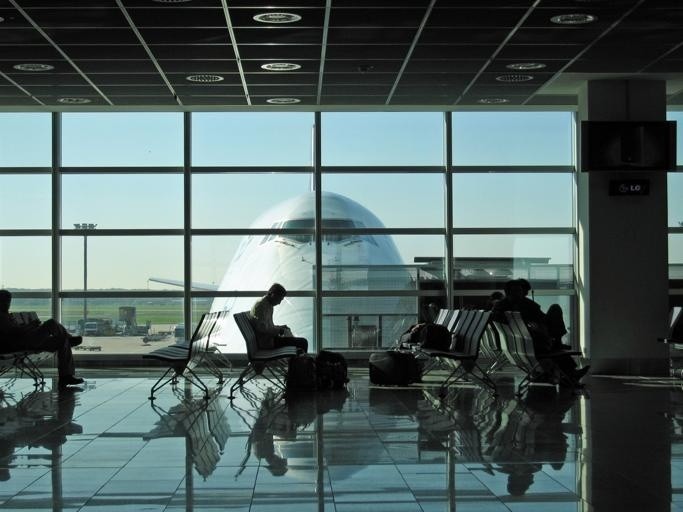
[
  {"x1": 0, "y1": 387, "x2": 83, "y2": 481},
  {"x1": 507, "y1": 386, "x2": 590, "y2": 497},
  {"x1": 490, "y1": 279, "x2": 590, "y2": 385},
  {"x1": 0, "y1": 290, "x2": 85, "y2": 387},
  {"x1": 250, "y1": 283, "x2": 308, "y2": 359},
  {"x1": 254, "y1": 432, "x2": 288, "y2": 476}
]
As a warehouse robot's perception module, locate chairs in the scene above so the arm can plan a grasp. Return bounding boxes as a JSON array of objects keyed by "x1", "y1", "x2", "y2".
[
  {"x1": 143, "y1": 385, "x2": 287, "y2": 482},
  {"x1": 0, "y1": 312, "x2": 297, "y2": 400},
  {"x1": 402, "y1": 305, "x2": 682, "y2": 401}
]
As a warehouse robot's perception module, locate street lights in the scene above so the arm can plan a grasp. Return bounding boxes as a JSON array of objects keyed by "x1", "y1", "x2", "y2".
[{"x1": 74, "y1": 223, "x2": 96, "y2": 322}]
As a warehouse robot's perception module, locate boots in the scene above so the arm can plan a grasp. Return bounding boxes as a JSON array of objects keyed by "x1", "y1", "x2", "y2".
[{"x1": 563, "y1": 365, "x2": 590, "y2": 384}]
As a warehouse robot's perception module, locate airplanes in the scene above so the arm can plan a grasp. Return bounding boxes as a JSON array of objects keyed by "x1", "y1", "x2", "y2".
[
  {"x1": 185, "y1": 370, "x2": 407, "y2": 492},
  {"x1": 147, "y1": 121, "x2": 418, "y2": 356}
]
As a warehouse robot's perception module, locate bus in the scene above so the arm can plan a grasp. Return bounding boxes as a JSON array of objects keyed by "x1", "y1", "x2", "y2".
[{"x1": 83, "y1": 321, "x2": 110, "y2": 336}]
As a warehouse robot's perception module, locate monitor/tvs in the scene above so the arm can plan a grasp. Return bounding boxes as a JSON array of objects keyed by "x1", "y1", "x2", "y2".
[{"x1": 581, "y1": 120, "x2": 677, "y2": 172}]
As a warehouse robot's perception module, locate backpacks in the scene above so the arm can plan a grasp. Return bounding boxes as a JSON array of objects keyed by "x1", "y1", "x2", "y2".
[
  {"x1": 286, "y1": 350, "x2": 347, "y2": 395},
  {"x1": 369, "y1": 351, "x2": 421, "y2": 386},
  {"x1": 402, "y1": 322, "x2": 452, "y2": 351}
]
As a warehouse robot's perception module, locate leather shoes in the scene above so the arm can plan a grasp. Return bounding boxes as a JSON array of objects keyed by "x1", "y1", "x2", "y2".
[
  {"x1": 58, "y1": 376, "x2": 84, "y2": 386},
  {"x1": 71, "y1": 336, "x2": 82, "y2": 346},
  {"x1": 58, "y1": 386, "x2": 84, "y2": 394}
]
